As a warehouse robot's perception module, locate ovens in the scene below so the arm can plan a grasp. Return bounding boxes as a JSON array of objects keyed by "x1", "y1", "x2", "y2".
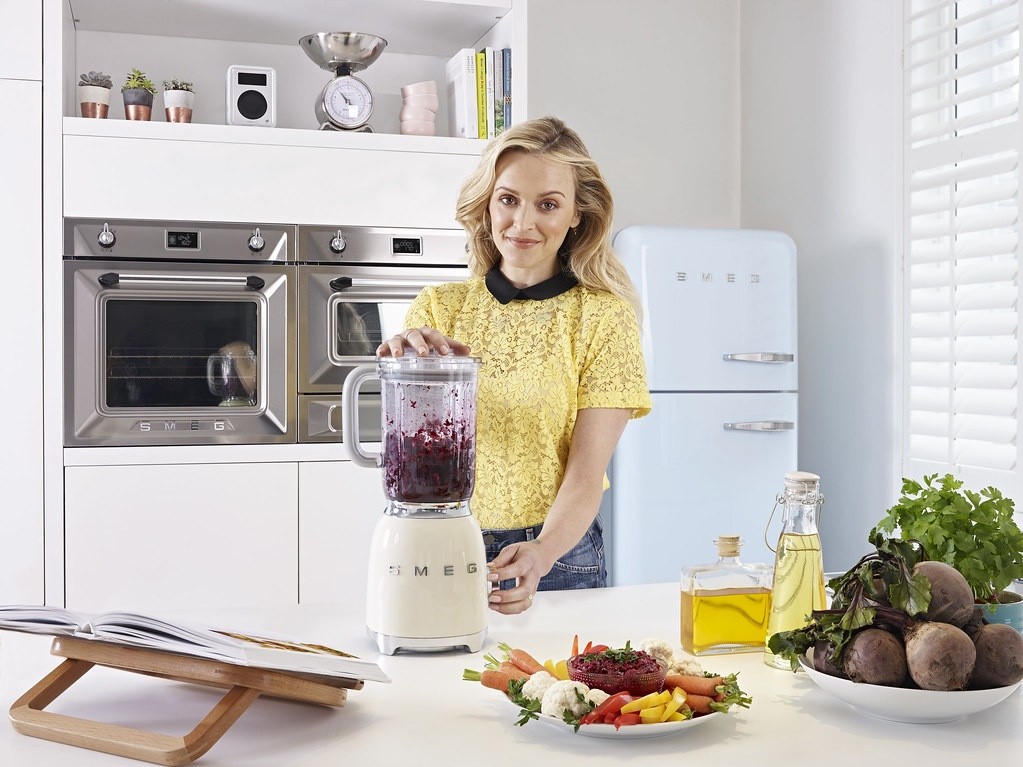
[{"x1": 65, "y1": 218, "x2": 482, "y2": 447}]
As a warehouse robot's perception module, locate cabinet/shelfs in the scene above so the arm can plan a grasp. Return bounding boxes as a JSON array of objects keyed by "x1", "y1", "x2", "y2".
[{"x1": 57, "y1": 442, "x2": 382, "y2": 605}]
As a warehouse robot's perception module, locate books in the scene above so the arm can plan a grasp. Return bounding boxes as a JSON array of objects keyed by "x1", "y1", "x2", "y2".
[
  {"x1": 1, "y1": 605, "x2": 392, "y2": 684},
  {"x1": 444, "y1": 48, "x2": 512, "y2": 140}
]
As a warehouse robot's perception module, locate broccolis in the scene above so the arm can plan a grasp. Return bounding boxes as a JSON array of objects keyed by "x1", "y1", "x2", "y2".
[
  {"x1": 520, "y1": 670, "x2": 610, "y2": 718},
  {"x1": 642, "y1": 639, "x2": 705, "y2": 677}
]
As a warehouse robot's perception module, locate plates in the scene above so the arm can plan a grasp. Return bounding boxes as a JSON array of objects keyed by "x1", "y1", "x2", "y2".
[
  {"x1": 505, "y1": 690, "x2": 726, "y2": 739},
  {"x1": 792, "y1": 651, "x2": 1023, "y2": 725}
]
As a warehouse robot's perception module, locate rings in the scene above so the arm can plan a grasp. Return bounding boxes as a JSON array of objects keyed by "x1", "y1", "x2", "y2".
[
  {"x1": 529, "y1": 595, "x2": 533, "y2": 600},
  {"x1": 405, "y1": 330, "x2": 415, "y2": 341}
]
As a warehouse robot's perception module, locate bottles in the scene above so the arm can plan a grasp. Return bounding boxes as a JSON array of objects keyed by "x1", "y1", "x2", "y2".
[
  {"x1": 681, "y1": 535, "x2": 774, "y2": 657},
  {"x1": 765, "y1": 473, "x2": 829, "y2": 673}
]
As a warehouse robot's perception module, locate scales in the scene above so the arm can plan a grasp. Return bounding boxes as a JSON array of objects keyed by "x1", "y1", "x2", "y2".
[{"x1": 300, "y1": 32, "x2": 389, "y2": 133}]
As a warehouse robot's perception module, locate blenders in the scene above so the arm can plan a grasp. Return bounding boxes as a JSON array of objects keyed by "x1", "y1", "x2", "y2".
[{"x1": 342, "y1": 347, "x2": 492, "y2": 656}]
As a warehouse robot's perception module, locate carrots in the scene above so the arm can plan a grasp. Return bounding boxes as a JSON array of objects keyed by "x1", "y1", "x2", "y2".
[
  {"x1": 480, "y1": 649, "x2": 559, "y2": 691},
  {"x1": 664, "y1": 674, "x2": 728, "y2": 714}
]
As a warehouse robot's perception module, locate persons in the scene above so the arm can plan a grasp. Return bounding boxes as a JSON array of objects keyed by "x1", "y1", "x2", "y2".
[
  {"x1": 375, "y1": 114, "x2": 651, "y2": 615},
  {"x1": 222, "y1": 342, "x2": 257, "y2": 397},
  {"x1": 336, "y1": 302, "x2": 372, "y2": 356}
]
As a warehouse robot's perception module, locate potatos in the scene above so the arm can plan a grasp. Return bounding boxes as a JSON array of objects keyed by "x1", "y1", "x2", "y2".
[{"x1": 810, "y1": 560, "x2": 1023, "y2": 692}]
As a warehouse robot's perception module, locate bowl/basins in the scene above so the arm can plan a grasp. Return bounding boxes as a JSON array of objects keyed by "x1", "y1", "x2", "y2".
[
  {"x1": 399, "y1": 80, "x2": 439, "y2": 135},
  {"x1": 568, "y1": 654, "x2": 669, "y2": 694}
]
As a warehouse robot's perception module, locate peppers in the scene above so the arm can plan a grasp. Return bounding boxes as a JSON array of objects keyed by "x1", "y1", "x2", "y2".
[
  {"x1": 545, "y1": 635, "x2": 608, "y2": 680},
  {"x1": 579, "y1": 686, "x2": 689, "y2": 730}
]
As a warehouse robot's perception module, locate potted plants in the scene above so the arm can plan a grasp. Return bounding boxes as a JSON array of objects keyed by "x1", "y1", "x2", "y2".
[
  {"x1": 75, "y1": 68, "x2": 113, "y2": 118},
  {"x1": 875, "y1": 472, "x2": 1023, "y2": 628},
  {"x1": 163, "y1": 75, "x2": 199, "y2": 122},
  {"x1": 119, "y1": 65, "x2": 157, "y2": 120}
]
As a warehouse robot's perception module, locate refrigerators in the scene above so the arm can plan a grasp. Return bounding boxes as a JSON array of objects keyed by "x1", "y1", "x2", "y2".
[{"x1": 595, "y1": 226, "x2": 800, "y2": 588}]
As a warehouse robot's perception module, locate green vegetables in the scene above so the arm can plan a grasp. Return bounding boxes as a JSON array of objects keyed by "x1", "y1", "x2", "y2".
[
  {"x1": 506, "y1": 677, "x2": 541, "y2": 726},
  {"x1": 583, "y1": 639, "x2": 640, "y2": 664},
  {"x1": 562, "y1": 686, "x2": 599, "y2": 734}
]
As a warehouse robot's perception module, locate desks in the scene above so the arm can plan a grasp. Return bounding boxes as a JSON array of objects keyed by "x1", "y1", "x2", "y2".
[{"x1": 0, "y1": 577, "x2": 1023, "y2": 767}]
